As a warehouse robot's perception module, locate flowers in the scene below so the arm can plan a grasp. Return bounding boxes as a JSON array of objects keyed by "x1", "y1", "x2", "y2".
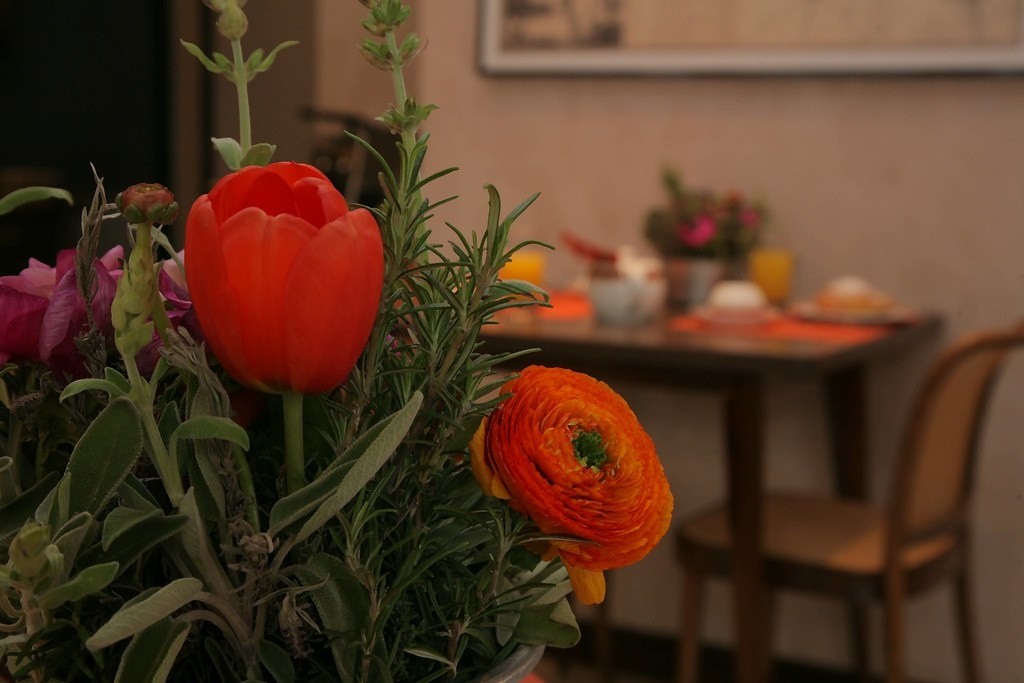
[
  {"x1": 642, "y1": 166, "x2": 775, "y2": 257},
  {"x1": 1, "y1": 0, "x2": 679, "y2": 683}
]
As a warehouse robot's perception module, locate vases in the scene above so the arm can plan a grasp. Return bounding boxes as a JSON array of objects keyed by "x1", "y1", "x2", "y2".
[{"x1": 666, "y1": 258, "x2": 728, "y2": 308}]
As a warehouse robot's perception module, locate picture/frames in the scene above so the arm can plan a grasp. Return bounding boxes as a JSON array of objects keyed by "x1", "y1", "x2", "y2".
[{"x1": 466, "y1": 0, "x2": 1024, "y2": 86}]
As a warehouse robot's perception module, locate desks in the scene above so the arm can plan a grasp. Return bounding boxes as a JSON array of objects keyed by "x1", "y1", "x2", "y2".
[{"x1": 450, "y1": 300, "x2": 949, "y2": 683}]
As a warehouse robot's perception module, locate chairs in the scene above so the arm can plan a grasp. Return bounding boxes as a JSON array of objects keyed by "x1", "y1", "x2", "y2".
[{"x1": 669, "y1": 330, "x2": 1024, "y2": 683}]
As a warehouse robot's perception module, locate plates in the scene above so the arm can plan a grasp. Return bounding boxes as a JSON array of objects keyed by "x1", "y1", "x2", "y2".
[
  {"x1": 792, "y1": 303, "x2": 920, "y2": 324},
  {"x1": 694, "y1": 306, "x2": 779, "y2": 325}
]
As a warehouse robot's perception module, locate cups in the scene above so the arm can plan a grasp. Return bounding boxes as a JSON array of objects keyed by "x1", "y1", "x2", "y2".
[
  {"x1": 591, "y1": 280, "x2": 646, "y2": 327},
  {"x1": 747, "y1": 229, "x2": 794, "y2": 308}
]
